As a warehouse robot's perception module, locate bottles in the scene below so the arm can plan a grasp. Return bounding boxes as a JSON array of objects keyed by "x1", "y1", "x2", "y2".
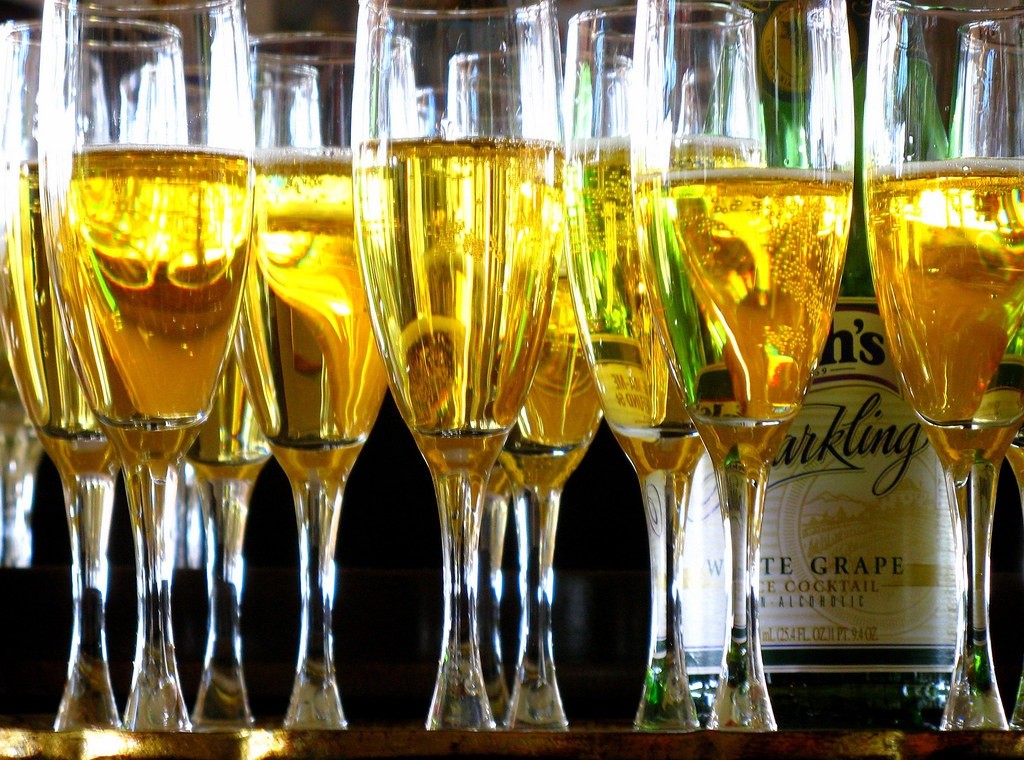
[
  {"x1": 640, "y1": 440, "x2": 753, "y2": 723},
  {"x1": 752, "y1": 3, "x2": 966, "y2": 730}
]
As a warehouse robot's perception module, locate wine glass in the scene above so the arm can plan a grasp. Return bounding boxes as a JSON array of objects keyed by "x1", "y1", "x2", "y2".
[{"x1": 0, "y1": 0, "x2": 1024, "y2": 729}]
